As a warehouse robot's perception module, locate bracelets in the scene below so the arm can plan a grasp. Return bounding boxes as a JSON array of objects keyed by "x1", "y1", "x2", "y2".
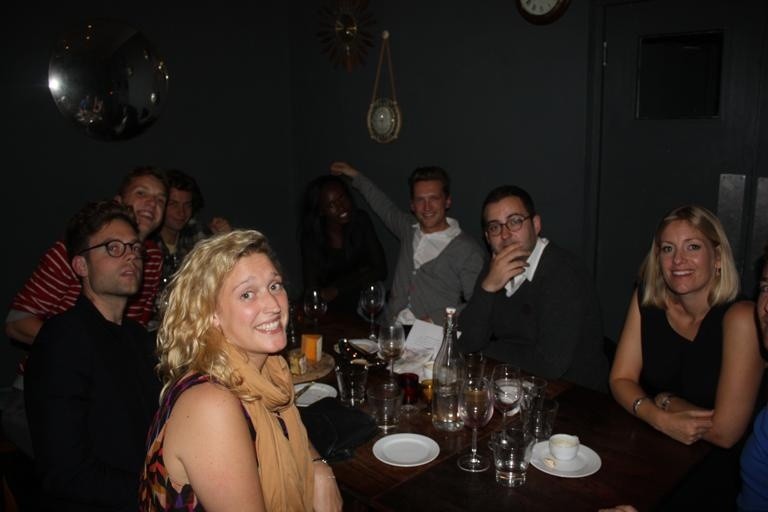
[
  {"x1": 309, "y1": 456, "x2": 327, "y2": 464},
  {"x1": 632, "y1": 395, "x2": 648, "y2": 415},
  {"x1": 313, "y1": 473, "x2": 337, "y2": 480}
]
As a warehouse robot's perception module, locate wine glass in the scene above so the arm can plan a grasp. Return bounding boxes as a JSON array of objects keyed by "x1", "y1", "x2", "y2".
[
  {"x1": 376, "y1": 319, "x2": 404, "y2": 377},
  {"x1": 362, "y1": 282, "x2": 384, "y2": 339},
  {"x1": 456, "y1": 377, "x2": 493, "y2": 473},
  {"x1": 489, "y1": 363, "x2": 523, "y2": 450}
]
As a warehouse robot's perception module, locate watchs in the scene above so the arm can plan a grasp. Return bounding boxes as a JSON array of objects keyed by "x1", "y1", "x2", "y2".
[{"x1": 660, "y1": 395, "x2": 679, "y2": 411}]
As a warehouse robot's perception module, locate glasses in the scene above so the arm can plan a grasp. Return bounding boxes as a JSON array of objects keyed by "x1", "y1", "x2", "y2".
[
  {"x1": 484, "y1": 214, "x2": 531, "y2": 238},
  {"x1": 78, "y1": 238, "x2": 143, "y2": 260}
]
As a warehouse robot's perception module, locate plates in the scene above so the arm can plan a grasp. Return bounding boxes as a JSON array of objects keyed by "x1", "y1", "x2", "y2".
[
  {"x1": 289, "y1": 381, "x2": 338, "y2": 408},
  {"x1": 286, "y1": 348, "x2": 335, "y2": 384},
  {"x1": 528, "y1": 441, "x2": 603, "y2": 479},
  {"x1": 371, "y1": 433, "x2": 440, "y2": 467}
]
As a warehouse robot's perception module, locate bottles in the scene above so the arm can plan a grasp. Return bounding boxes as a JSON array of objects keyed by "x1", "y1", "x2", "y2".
[{"x1": 430, "y1": 305, "x2": 466, "y2": 432}]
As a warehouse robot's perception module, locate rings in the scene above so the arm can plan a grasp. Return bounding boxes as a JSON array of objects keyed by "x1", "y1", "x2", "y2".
[
  {"x1": 698, "y1": 435, "x2": 704, "y2": 441},
  {"x1": 623, "y1": 504, "x2": 629, "y2": 510}
]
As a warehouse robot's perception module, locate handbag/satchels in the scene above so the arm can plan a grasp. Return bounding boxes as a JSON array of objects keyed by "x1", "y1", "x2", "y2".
[{"x1": 300, "y1": 396, "x2": 376, "y2": 462}]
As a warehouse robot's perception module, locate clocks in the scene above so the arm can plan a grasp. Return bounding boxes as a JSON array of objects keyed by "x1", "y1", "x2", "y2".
[{"x1": 517, "y1": 0, "x2": 569, "y2": 24}]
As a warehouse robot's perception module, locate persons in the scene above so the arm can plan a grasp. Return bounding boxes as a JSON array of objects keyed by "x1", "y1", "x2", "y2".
[
  {"x1": 296, "y1": 174, "x2": 388, "y2": 326},
  {"x1": 608, "y1": 204, "x2": 764, "y2": 450},
  {"x1": 2, "y1": 167, "x2": 168, "y2": 465},
  {"x1": 23, "y1": 201, "x2": 163, "y2": 510},
  {"x1": 148, "y1": 175, "x2": 232, "y2": 333},
  {"x1": 137, "y1": 230, "x2": 344, "y2": 511},
  {"x1": 456, "y1": 185, "x2": 609, "y2": 394},
  {"x1": 330, "y1": 159, "x2": 484, "y2": 333},
  {"x1": 598, "y1": 254, "x2": 766, "y2": 511}
]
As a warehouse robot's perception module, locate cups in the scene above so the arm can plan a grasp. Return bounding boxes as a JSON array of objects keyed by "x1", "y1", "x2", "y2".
[
  {"x1": 467, "y1": 351, "x2": 488, "y2": 377},
  {"x1": 303, "y1": 287, "x2": 327, "y2": 335},
  {"x1": 334, "y1": 365, "x2": 368, "y2": 407},
  {"x1": 367, "y1": 383, "x2": 401, "y2": 429},
  {"x1": 420, "y1": 378, "x2": 460, "y2": 415},
  {"x1": 493, "y1": 375, "x2": 580, "y2": 486},
  {"x1": 398, "y1": 373, "x2": 420, "y2": 407}
]
